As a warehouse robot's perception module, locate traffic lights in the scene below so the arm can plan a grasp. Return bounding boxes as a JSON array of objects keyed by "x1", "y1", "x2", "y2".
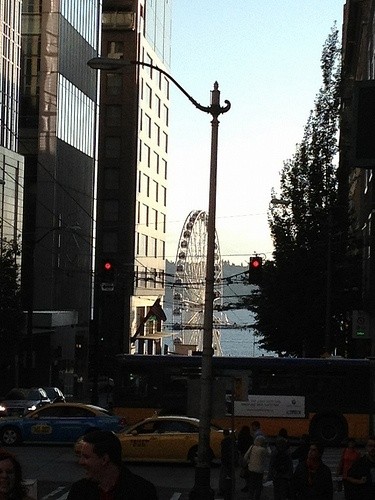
[
  {"x1": 98, "y1": 256, "x2": 114, "y2": 284},
  {"x1": 248, "y1": 256, "x2": 281, "y2": 286}
]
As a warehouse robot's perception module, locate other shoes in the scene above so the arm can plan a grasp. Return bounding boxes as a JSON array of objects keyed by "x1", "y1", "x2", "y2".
[
  {"x1": 240, "y1": 488, "x2": 248, "y2": 492},
  {"x1": 262, "y1": 480, "x2": 274, "y2": 488}
]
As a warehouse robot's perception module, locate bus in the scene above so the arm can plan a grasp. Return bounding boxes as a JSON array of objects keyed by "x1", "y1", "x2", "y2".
[{"x1": 102, "y1": 352, "x2": 375, "y2": 450}]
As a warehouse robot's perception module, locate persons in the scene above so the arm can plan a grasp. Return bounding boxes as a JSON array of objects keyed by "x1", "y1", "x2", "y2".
[
  {"x1": 240, "y1": 421, "x2": 263, "y2": 492},
  {"x1": 295, "y1": 441, "x2": 333, "y2": 500},
  {"x1": 269, "y1": 438, "x2": 295, "y2": 500},
  {"x1": 339, "y1": 437, "x2": 360, "y2": 483},
  {"x1": 342, "y1": 439, "x2": 375, "y2": 500},
  {"x1": 243, "y1": 435, "x2": 268, "y2": 500},
  {"x1": 0, "y1": 451, "x2": 35, "y2": 500},
  {"x1": 67, "y1": 431, "x2": 157, "y2": 500}
]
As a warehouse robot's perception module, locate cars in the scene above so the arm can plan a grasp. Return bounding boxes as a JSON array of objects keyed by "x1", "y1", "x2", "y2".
[
  {"x1": 0, "y1": 397, "x2": 112, "y2": 447},
  {"x1": 115, "y1": 411, "x2": 229, "y2": 467}
]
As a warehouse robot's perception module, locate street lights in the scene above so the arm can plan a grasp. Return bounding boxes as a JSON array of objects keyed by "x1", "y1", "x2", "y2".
[{"x1": 87, "y1": 55, "x2": 231, "y2": 500}]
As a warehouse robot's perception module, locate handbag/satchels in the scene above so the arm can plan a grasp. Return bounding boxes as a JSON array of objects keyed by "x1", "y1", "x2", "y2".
[
  {"x1": 335, "y1": 463, "x2": 344, "y2": 476},
  {"x1": 241, "y1": 445, "x2": 253, "y2": 465}
]
